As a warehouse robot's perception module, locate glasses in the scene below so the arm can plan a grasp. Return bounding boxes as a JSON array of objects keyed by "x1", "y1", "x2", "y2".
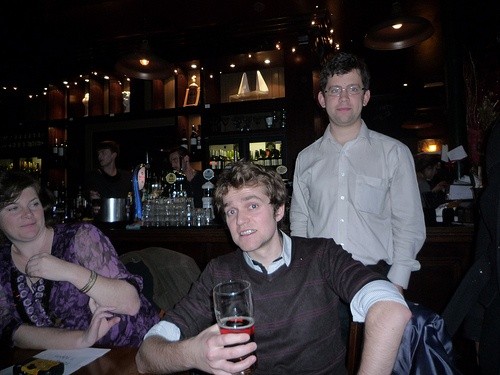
[{"x1": 323, "y1": 86, "x2": 368, "y2": 97}]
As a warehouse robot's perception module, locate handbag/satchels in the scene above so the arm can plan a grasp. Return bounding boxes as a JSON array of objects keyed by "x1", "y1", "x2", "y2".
[{"x1": 390, "y1": 308, "x2": 460, "y2": 374}]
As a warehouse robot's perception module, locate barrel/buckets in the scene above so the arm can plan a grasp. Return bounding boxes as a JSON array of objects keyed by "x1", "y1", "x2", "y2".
[{"x1": 100, "y1": 196, "x2": 130, "y2": 225}]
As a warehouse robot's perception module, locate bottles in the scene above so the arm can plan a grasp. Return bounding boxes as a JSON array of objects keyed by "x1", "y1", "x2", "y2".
[
  {"x1": 127, "y1": 192, "x2": 134, "y2": 213},
  {"x1": 209, "y1": 146, "x2": 240, "y2": 172},
  {"x1": 1, "y1": 136, "x2": 66, "y2": 218},
  {"x1": 252, "y1": 148, "x2": 282, "y2": 170},
  {"x1": 190, "y1": 124, "x2": 204, "y2": 155}
]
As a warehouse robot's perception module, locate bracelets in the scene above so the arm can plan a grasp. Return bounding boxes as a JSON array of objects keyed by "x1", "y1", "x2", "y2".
[{"x1": 78, "y1": 270, "x2": 98, "y2": 294}]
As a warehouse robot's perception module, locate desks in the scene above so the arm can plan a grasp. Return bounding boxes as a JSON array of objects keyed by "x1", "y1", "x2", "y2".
[{"x1": 0, "y1": 341, "x2": 140, "y2": 375}]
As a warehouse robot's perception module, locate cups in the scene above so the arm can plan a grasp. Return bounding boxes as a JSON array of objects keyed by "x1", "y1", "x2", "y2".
[
  {"x1": 212, "y1": 108, "x2": 286, "y2": 132},
  {"x1": 142, "y1": 182, "x2": 216, "y2": 227}
]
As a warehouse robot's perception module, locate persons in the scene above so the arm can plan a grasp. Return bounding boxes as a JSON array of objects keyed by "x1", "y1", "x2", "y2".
[
  {"x1": 0, "y1": 168, "x2": 159, "y2": 349},
  {"x1": 426, "y1": 173, "x2": 473, "y2": 226},
  {"x1": 135, "y1": 158, "x2": 412, "y2": 375},
  {"x1": 290, "y1": 52, "x2": 426, "y2": 295},
  {"x1": 465, "y1": 119, "x2": 500, "y2": 375},
  {"x1": 81, "y1": 141, "x2": 127, "y2": 214},
  {"x1": 159, "y1": 146, "x2": 214, "y2": 208}
]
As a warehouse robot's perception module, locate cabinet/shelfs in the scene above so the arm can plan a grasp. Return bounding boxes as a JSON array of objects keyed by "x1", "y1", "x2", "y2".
[{"x1": 0, "y1": 11, "x2": 315, "y2": 181}]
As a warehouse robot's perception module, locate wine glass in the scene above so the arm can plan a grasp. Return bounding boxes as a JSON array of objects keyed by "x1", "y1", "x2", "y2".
[{"x1": 213, "y1": 280, "x2": 253, "y2": 375}]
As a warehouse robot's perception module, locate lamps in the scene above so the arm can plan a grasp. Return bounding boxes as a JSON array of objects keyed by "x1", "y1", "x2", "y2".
[{"x1": 363, "y1": 0, "x2": 436, "y2": 51}]
